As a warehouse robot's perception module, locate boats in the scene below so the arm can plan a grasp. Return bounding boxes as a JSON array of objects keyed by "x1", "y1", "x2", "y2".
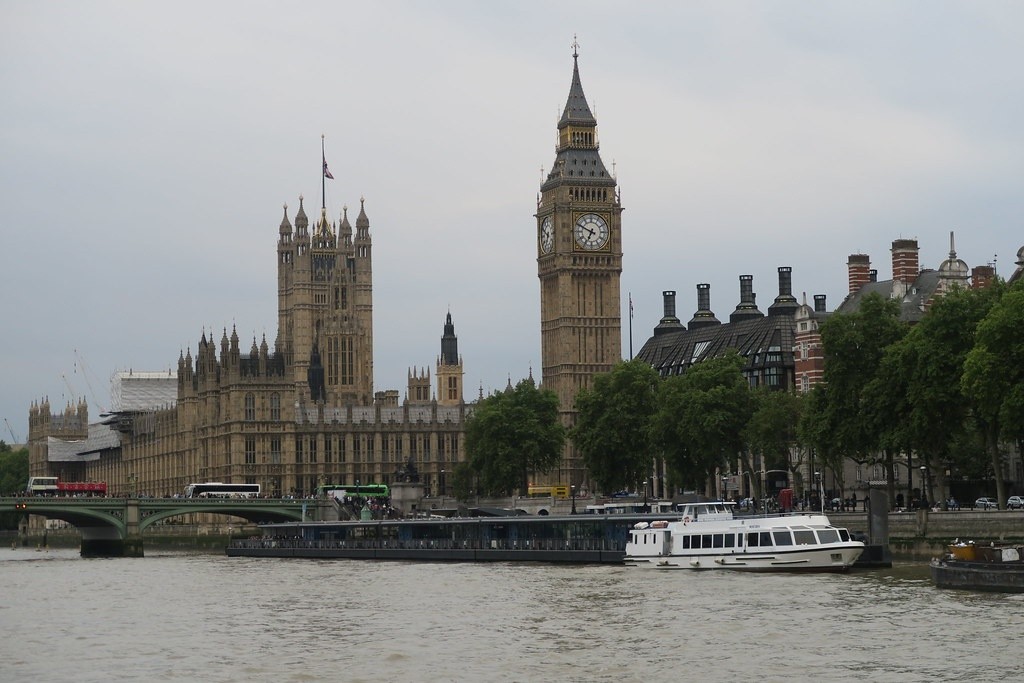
[
  {"x1": 613, "y1": 491, "x2": 628, "y2": 498},
  {"x1": 621, "y1": 502, "x2": 866, "y2": 574}
]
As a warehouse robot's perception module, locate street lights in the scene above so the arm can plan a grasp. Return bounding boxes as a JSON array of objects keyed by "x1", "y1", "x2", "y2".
[
  {"x1": 569, "y1": 484, "x2": 577, "y2": 515},
  {"x1": 722, "y1": 475, "x2": 729, "y2": 503},
  {"x1": 919, "y1": 466, "x2": 928, "y2": 510},
  {"x1": 812, "y1": 471, "x2": 820, "y2": 512}
]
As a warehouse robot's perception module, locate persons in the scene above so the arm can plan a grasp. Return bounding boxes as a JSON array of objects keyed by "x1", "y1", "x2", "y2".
[
  {"x1": 9, "y1": 488, "x2": 283, "y2": 501},
  {"x1": 950, "y1": 495, "x2": 957, "y2": 512},
  {"x1": 345, "y1": 493, "x2": 397, "y2": 520},
  {"x1": 895, "y1": 489, "x2": 929, "y2": 515}
]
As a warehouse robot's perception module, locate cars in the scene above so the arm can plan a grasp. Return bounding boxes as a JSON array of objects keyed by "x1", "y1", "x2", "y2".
[
  {"x1": 975, "y1": 496, "x2": 999, "y2": 510},
  {"x1": 1007, "y1": 495, "x2": 1024, "y2": 510},
  {"x1": 830, "y1": 497, "x2": 856, "y2": 508}
]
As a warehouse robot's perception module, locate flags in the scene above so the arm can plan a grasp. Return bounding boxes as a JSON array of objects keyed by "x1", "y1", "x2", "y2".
[{"x1": 323, "y1": 152, "x2": 334, "y2": 179}]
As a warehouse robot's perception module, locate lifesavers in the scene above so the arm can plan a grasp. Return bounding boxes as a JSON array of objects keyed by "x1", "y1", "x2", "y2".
[{"x1": 684, "y1": 517, "x2": 690, "y2": 522}]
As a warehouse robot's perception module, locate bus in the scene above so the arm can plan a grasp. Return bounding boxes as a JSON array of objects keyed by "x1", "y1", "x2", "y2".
[
  {"x1": 310, "y1": 483, "x2": 390, "y2": 504},
  {"x1": 527, "y1": 483, "x2": 568, "y2": 498},
  {"x1": 182, "y1": 482, "x2": 262, "y2": 503}
]
